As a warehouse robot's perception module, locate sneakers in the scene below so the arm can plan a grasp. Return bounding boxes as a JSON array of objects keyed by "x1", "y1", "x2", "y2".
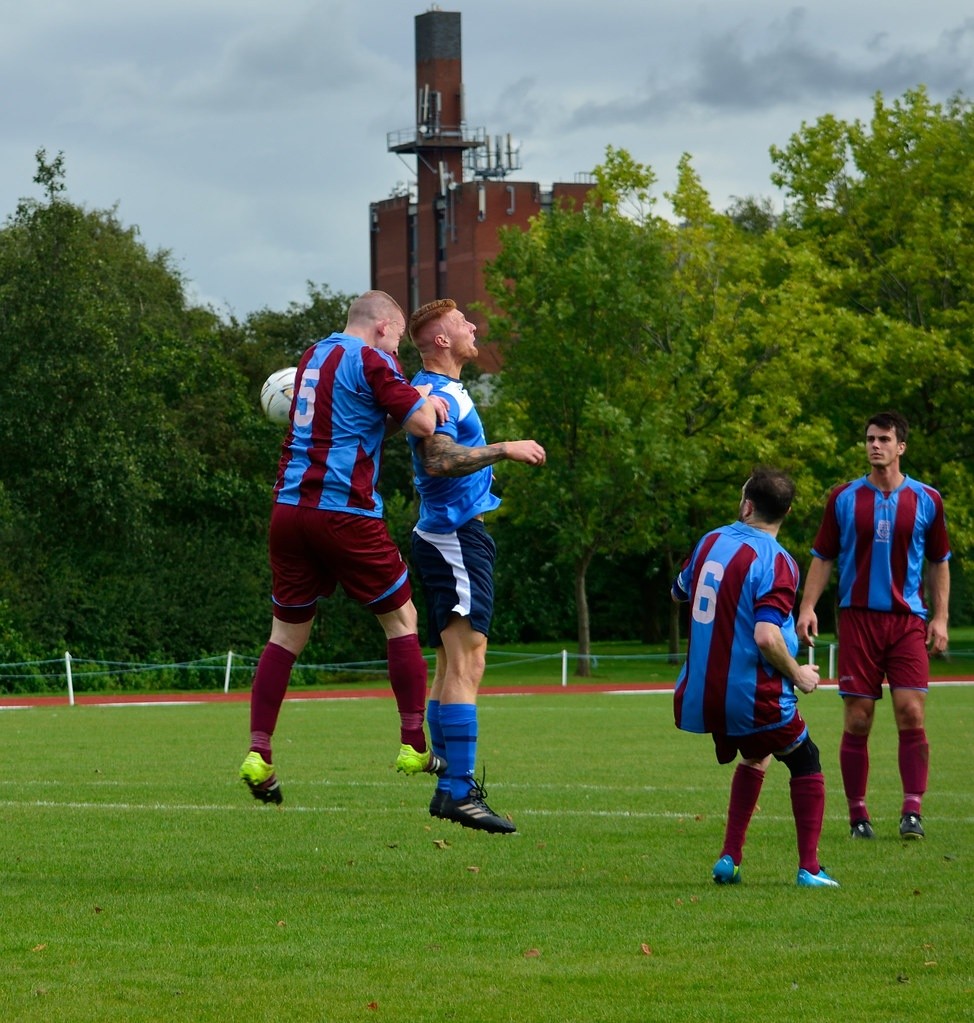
[
  {"x1": 898, "y1": 813, "x2": 925, "y2": 840},
  {"x1": 393, "y1": 741, "x2": 446, "y2": 775},
  {"x1": 712, "y1": 854, "x2": 740, "y2": 884},
  {"x1": 851, "y1": 818, "x2": 874, "y2": 839},
  {"x1": 237, "y1": 750, "x2": 283, "y2": 808},
  {"x1": 429, "y1": 785, "x2": 516, "y2": 834},
  {"x1": 795, "y1": 866, "x2": 840, "y2": 889}
]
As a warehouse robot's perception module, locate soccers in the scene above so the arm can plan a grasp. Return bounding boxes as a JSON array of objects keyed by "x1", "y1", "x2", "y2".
[{"x1": 261, "y1": 366, "x2": 300, "y2": 424}]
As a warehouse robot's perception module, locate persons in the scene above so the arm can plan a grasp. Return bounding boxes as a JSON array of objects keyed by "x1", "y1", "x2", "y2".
[
  {"x1": 796, "y1": 410, "x2": 951, "y2": 840},
  {"x1": 671, "y1": 469, "x2": 844, "y2": 886},
  {"x1": 238, "y1": 289, "x2": 450, "y2": 804},
  {"x1": 402, "y1": 298, "x2": 547, "y2": 834}
]
